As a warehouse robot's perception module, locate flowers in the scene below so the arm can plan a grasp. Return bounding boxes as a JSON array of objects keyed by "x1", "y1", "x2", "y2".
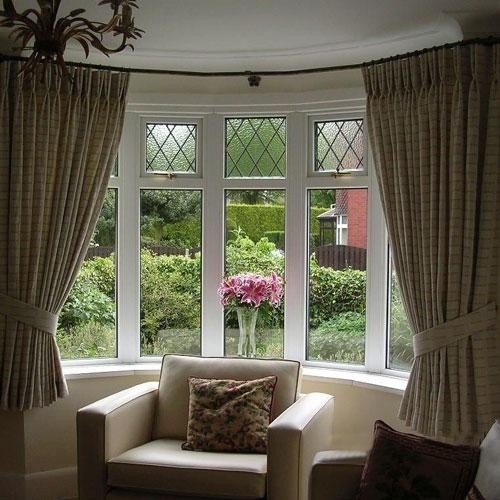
[{"x1": 218, "y1": 271, "x2": 285, "y2": 357}]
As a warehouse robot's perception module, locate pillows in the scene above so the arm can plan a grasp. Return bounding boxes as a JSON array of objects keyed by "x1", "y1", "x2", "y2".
[
  {"x1": 358, "y1": 420, "x2": 481, "y2": 500},
  {"x1": 182, "y1": 375, "x2": 278, "y2": 455}
]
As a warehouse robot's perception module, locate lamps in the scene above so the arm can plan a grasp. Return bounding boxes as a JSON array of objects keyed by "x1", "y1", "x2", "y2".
[{"x1": 0, "y1": 1, "x2": 147, "y2": 88}]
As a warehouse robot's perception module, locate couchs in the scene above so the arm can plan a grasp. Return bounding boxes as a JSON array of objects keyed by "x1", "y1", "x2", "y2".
[
  {"x1": 75, "y1": 352, "x2": 335, "y2": 500},
  {"x1": 308, "y1": 421, "x2": 499, "y2": 499}
]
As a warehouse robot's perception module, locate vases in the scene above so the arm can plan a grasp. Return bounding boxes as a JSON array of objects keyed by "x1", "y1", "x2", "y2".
[{"x1": 236, "y1": 307, "x2": 258, "y2": 359}]
